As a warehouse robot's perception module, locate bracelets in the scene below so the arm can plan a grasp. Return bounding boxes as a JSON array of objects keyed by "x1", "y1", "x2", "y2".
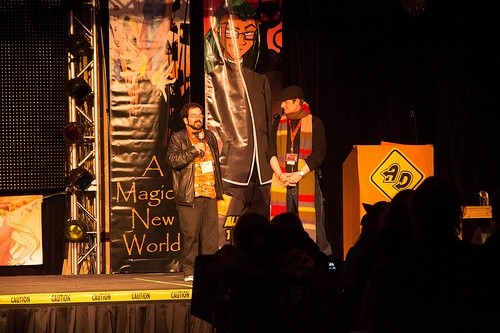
[{"x1": 279, "y1": 173, "x2": 282, "y2": 179}]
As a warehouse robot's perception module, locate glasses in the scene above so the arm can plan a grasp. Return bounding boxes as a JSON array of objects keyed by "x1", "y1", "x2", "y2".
[{"x1": 188, "y1": 114, "x2": 204, "y2": 119}]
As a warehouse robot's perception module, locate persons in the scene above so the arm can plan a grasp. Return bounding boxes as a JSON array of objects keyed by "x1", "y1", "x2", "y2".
[
  {"x1": 266, "y1": 85, "x2": 336, "y2": 269},
  {"x1": 203, "y1": 172, "x2": 500, "y2": 333},
  {"x1": 167, "y1": 103, "x2": 225, "y2": 281}
]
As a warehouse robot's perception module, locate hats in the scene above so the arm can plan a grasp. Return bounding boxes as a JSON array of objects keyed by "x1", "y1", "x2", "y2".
[{"x1": 274, "y1": 86, "x2": 304, "y2": 101}]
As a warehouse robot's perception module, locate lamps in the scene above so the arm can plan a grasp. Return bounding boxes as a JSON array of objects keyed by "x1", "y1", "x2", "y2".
[
  {"x1": 62, "y1": 219, "x2": 89, "y2": 243},
  {"x1": 66, "y1": 165, "x2": 96, "y2": 192},
  {"x1": 64, "y1": 32, "x2": 93, "y2": 62},
  {"x1": 64, "y1": 77, "x2": 92, "y2": 104},
  {"x1": 61, "y1": 120, "x2": 85, "y2": 146}
]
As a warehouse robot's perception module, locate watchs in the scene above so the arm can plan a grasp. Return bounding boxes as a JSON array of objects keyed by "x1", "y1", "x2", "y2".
[{"x1": 299, "y1": 170, "x2": 305, "y2": 177}]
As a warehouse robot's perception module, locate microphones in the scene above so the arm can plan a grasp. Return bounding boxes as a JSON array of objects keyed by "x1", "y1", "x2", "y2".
[{"x1": 199, "y1": 132, "x2": 204, "y2": 157}]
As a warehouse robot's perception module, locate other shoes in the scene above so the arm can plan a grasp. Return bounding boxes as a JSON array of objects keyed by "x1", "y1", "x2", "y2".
[{"x1": 184, "y1": 274, "x2": 194, "y2": 282}]
